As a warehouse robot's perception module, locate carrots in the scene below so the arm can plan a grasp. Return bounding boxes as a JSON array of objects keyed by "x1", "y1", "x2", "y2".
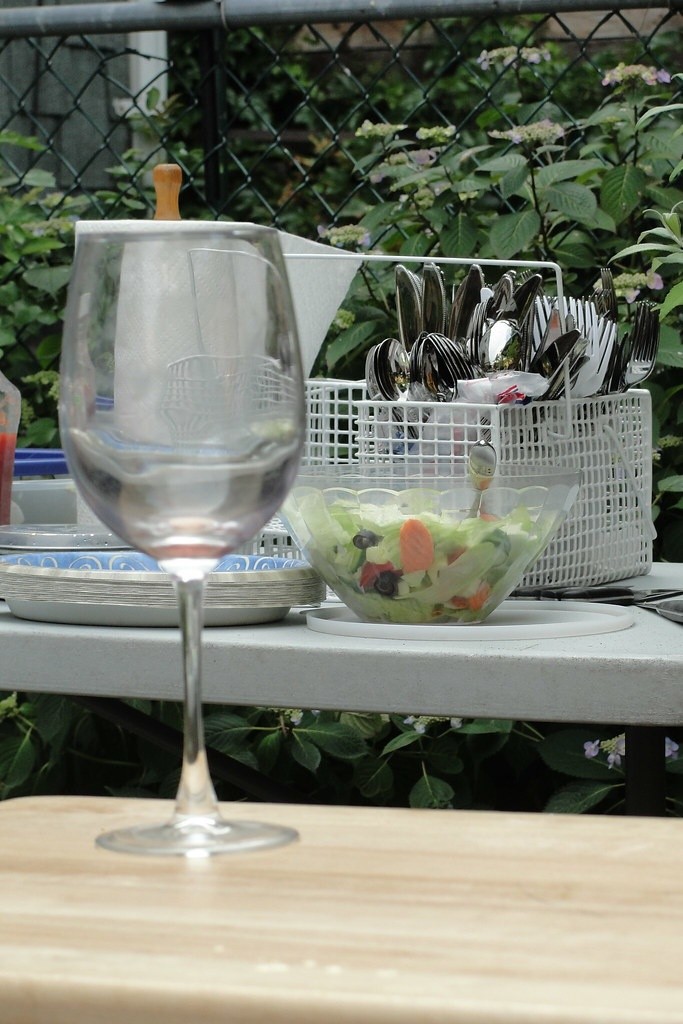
[{"x1": 401, "y1": 518, "x2": 433, "y2": 572}]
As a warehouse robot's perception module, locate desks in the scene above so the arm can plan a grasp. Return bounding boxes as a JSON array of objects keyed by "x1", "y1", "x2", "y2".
[
  {"x1": 0, "y1": 794, "x2": 683, "y2": 1024},
  {"x1": 0, "y1": 562, "x2": 683, "y2": 725}
]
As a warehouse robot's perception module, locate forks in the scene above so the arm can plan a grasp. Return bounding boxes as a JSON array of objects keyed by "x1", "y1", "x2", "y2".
[{"x1": 520, "y1": 268, "x2": 660, "y2": 434}]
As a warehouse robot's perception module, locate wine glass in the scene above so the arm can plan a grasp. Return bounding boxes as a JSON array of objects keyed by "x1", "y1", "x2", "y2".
[{"x1": 54, "y1": 221, "x2": 299, "y2": 858}]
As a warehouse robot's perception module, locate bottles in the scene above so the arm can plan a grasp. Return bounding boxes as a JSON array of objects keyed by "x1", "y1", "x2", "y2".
[{"x1": 0, "y1": 372, "x2": 21, "y2": 527}]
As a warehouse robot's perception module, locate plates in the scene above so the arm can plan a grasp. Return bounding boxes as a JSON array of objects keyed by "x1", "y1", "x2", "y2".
[{"x1": 1, "y1": 550, "x2": 326, "y2": 627}]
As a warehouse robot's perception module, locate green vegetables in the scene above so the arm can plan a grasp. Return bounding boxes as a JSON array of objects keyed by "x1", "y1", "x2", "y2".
[{"x1": 287, "y1": 501, "x2": 541, "y2": 624}]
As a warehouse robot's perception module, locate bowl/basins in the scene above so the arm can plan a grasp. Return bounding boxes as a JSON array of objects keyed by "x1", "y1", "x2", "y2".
[{"x1": 275, "y1": 464, "x2": 582, "y2": 625}]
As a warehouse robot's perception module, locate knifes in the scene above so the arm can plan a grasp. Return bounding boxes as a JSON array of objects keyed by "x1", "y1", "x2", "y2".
[
  {"x1": 634, "y1": 600, "x2": 683, "y2": 623},
  {"x1": 510, "y1": 583, "x2": 683, "y2": 603},
  {"x1": 394, "y1": 262, "x2": 590, "y2": 449}
]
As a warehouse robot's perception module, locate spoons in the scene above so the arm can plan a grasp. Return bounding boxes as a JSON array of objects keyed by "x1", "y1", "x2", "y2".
[{"x1": 367, "y1": 332, "x2": 476, "y2": 457}]
